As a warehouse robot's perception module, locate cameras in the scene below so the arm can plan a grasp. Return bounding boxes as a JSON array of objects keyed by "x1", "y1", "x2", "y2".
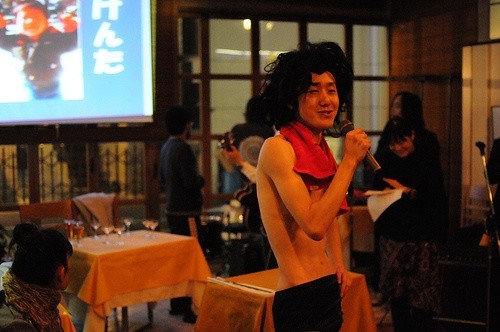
[{"x1": 217, "y1": 132, "x2": 239, "y2": 152}]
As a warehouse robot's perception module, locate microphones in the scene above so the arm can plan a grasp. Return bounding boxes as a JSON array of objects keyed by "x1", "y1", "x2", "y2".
[{"x1": 339, "y1": 119, "x2": 382, "y2": 172}]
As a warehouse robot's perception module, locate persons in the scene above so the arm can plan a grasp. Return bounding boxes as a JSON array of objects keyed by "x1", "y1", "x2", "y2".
[
  {"x1": 254, "y1": 41, "x2": 372, "y2": 332},
  {"x1": 0, "y1": 90, "x2": 500, "y2": 332},
  {"x1": 0, "y1": 0, "x2": 78, "y2": 100}
]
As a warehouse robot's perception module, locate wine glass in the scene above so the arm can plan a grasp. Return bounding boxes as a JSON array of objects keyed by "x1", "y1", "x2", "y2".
[
  {"x1": 72, "y1": 221, "x2": 86, "y2": 248},
  {"x1": 124, "y1": 217, "x2": 133, "y2": 235},
  {"x1": 101, "y1": 226, "x2": 113, "y2": 245},
  {"x1": 64, "y1": 219, "x2": 75, "y2": 245},
  {"x1": 90, "y1": 221, "x2": 101, "y2": 240},
  {"x1": 113, "y1": 226, "x2": 125, "y2": 245},
  {"x1": 142, "y1": 219, "x2": 158, "y2": 239}
]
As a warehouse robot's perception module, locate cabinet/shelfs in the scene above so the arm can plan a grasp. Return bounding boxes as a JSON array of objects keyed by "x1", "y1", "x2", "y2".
[{"x1": 432, "y1": 238, "x2": 496, "y2": 332}]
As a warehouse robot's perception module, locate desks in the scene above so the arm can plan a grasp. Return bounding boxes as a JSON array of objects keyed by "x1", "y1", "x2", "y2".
[
  {"x1": 193, "y1": 267, "x2": 377, "y2": 332},
  {"x1": 350, "y1": 206, "x2": 375, "y2": 254},
  {"x1": 61, "y1": 230, "x2": 212, "y2": 332}
]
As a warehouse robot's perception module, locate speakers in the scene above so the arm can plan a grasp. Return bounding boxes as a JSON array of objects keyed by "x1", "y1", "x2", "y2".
[{"x1": 430, "y1": 238, "x2": 497, "y2": 332}]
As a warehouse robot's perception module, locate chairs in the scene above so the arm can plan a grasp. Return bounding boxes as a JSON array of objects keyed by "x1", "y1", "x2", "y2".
[
  {"x1": 72, "y1": 193, "x2": 120, "y2": 237},
  {"x1": 18, "y1": 199, "x2": 72, "y2": 239},
  {"x1": 187, "y1": 214, "x2": 230, "y2": 277}
]
